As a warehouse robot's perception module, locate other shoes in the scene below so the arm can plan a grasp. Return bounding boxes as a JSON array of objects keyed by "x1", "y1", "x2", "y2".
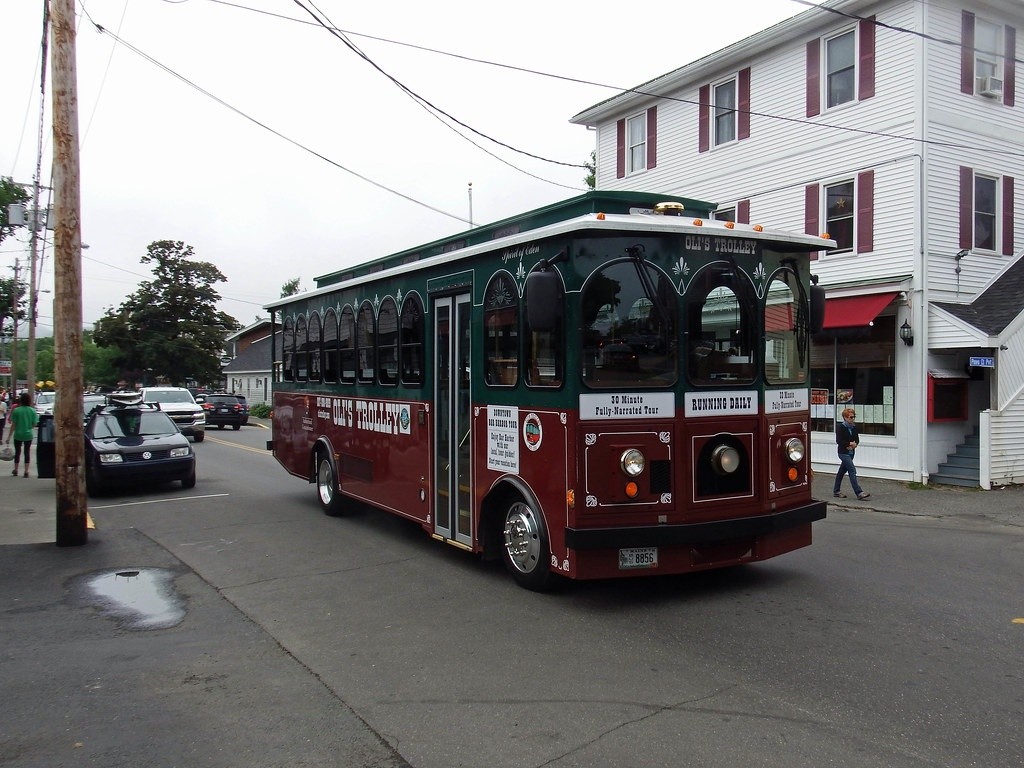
[
  {"x1": 12, "y1": 470, "x2": 19, "y2": 476},
  {"x1": 24, "y1": 472, "x2": 29, "y2": 477}
]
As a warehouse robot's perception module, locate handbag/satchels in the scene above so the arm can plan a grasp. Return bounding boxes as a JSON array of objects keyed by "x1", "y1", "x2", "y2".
[{"x1": 0, "y1": 443, "x2": 15, "y2": 461}]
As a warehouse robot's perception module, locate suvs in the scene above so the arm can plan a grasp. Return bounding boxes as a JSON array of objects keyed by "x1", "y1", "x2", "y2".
[
  {"x1": 139, "y1": 387, "x2": 206, "y2": 442},
  {"x1": 36, "y1": 390, "x2": 196, "y2": 495},
  {"x1": 196, "y1": 393, "x2": 242, "y2": 430},
  {"x1": 188, "y1": 386, "x2": 249, "y2": 425}
]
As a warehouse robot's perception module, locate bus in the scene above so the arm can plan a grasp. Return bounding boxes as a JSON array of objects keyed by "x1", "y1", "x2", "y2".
[{"x1": 264, "y1": 192, "x2": 839, "y2": 594}]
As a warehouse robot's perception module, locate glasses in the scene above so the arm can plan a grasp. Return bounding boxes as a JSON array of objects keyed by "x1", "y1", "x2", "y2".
[{"x1": 847, "y1": 416, "x2": 856, "y2": 419}]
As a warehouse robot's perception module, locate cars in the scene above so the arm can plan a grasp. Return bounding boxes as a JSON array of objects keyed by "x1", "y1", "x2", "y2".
[
  {"x1": 624, "y1": 335, "x2": 650, "y2": 354},
  {"x1": 600, "y1": 343, "x2": 637, "y2": 371},
  {"x1": 34, "y1": 390, "x2": 143, "y2": 427}
]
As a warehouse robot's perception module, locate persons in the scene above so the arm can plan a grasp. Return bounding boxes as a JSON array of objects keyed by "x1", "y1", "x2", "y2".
[
  {"x1": 0, "y1": 387, "x2": 40, "y2": 477},
  {"x1": 833, "y1": 408, "x2": 870, "y2": 500}
]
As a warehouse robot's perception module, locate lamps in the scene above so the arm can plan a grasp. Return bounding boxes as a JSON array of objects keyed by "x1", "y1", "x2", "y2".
[
  {"x1": 899, "y1": 317, "x2": 914, "y2": 347},
  {"x1": 955, "y1": 249, "x2": 970, "y2": 273}
]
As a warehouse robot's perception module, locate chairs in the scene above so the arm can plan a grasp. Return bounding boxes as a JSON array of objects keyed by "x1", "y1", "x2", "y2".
[{"x1": 816, "y1": 393, "x2": 834, "y2": 432}]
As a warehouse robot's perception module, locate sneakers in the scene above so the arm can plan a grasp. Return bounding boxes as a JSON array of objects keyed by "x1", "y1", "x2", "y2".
[
  {"x1": 857, "y1": 492, "x2": 870, "y2": 500},
  {"x1": 834, "y1": 492, "x2": 846, "y2": 498}
]
